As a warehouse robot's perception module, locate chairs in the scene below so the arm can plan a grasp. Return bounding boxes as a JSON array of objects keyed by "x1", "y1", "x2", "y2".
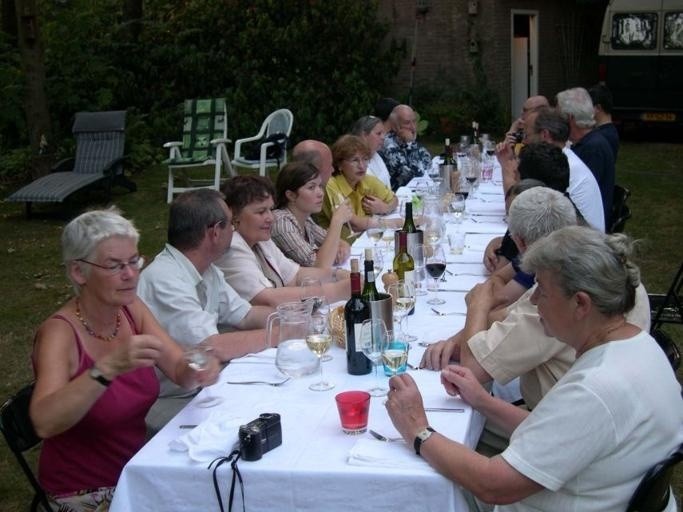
[
  {"x1": 230, "y1": 108, "x2": 293, "y2": 177},
  {"x1": 7, "y1": 110, "x2": 137, "y2": 221},
  {"x1": 0, "y1": 379, "x2": 52, "y2": 512},
  {"x1": 606, "y1": 186, "x2": 683, "y2": 512},
  {"x1": 160, "y1": 96, "x2": 237, "y2": 206}
]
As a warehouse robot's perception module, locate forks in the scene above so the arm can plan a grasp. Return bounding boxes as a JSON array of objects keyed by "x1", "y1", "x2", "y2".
[
  {"x1": 227, "y1": 377, "x2": 290, "y2": 387},
  {"x1": 371, "y1": 429, "x2": 404, "y2": 443}
]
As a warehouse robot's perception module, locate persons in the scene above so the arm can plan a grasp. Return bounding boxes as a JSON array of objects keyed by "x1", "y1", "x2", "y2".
[
  {"x1": 30, "y1": 209, "x2": 220, "y2": 512},
  {"x1": 419, "y1": 87, "x2": 652, "y2": 510},
  {"x1": 385, "y1": 225, "x2": 682, "y2": 511},
  {"x1": 135, "y1": 103, "x2": 432, "y2": 431}
]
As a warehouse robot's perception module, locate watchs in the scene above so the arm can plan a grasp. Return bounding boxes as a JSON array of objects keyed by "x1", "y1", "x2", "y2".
[
  {"x1": 414, "y1": 427, "x2": 435, "y2": 456},
  {"x1": 89, "y1": 365, "x2": 112, "y2": 386}
]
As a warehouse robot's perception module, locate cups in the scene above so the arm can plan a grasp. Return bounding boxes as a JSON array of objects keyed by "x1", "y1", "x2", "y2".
[
  {"x1": 336, "y1": 391, "x2": 371, "y2": 434},
  {"x1": 265, "y1": 297, "x2": 320, "y2": 377}
]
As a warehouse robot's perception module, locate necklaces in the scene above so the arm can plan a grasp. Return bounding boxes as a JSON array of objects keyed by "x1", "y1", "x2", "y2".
[{"x1": 75, "y1": 296, "x2": 121, "y2": 341}]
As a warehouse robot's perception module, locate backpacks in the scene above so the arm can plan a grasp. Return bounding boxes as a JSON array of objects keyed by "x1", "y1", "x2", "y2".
[{"x1": 242, "y1": 133, "x2": 289, "y2": 160}]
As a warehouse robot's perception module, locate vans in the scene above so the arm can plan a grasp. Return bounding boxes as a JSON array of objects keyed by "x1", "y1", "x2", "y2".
[{"x1": 595, "y1": 0, "x2": 683, "y2": 137}]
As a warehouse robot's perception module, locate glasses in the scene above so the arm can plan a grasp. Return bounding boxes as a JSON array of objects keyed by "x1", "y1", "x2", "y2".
[{"x1": 75, "y1": 255, "x2": 148, "y2": 275}]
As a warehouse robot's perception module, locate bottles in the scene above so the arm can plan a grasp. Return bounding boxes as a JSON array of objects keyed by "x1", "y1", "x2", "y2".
[{"x1": 345, "y1": 121, "x2": 484, "y2": 376}]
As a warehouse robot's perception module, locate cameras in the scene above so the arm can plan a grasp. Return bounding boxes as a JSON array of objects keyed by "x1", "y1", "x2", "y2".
[
  {"x1": 512, "y1": 129, "x2": 525, "y2": 143},
  {"x1": 238, "y1": 413, "x2": 282, "y2": 461}
]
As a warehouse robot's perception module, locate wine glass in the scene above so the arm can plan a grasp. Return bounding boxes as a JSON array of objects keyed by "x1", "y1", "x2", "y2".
[
  {"x1": 387, "y1": 279, "x2": 409, "y2": 342},
  {"x1": 301, "y1": 134, "x2": 496, "y2": 286},
  {"x1": 305, "y1": 298, "x2": 333, "y2": 363},
  {"x1": 302, "y1": 276, "x2": 324, "y2": 320},
  {"x1": 382, "y1": 329, "x2": 410, "y2": 391},
  {"x1": 180, "y1": 326, "x2": 223, "y2": 409},
  {"x1": 359, "y1": 317, "x2": 391, "y2": 398},
  {"x1": 399, "y1": 280, "x2": 417, "y2": 342}
]
